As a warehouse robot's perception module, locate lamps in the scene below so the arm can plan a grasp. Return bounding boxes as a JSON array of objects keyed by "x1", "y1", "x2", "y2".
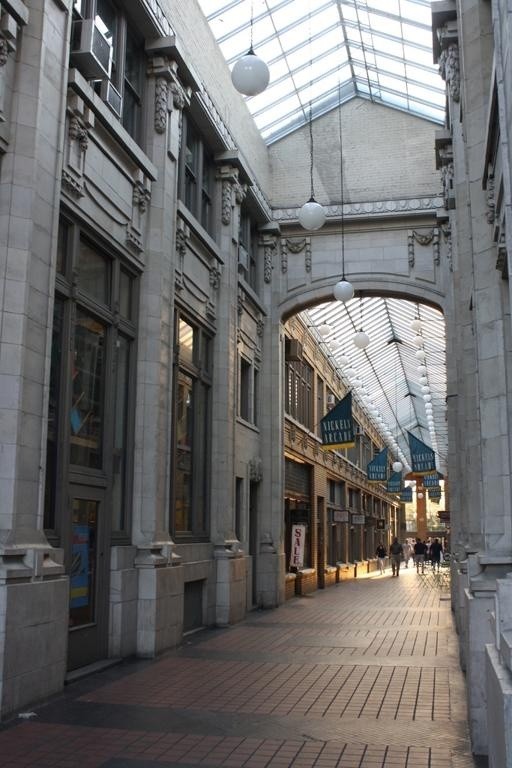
[
  {"x1": 229, "y1": 0, "x2": 271, "y2": 98},
  {"x1": 330, "y1": 65, "x2": 355, "y2": 303},
  {"x1": 307, "y1": 297, "x2": 444, "y2": 499},
  {"x1": 296, "y1": 2, "x2": 326, "y2": 230}
]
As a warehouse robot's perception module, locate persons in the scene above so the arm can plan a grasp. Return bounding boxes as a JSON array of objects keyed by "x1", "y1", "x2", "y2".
[
  {"x1": 403, "y1": 537, "x2": 445, "y2": 575},
  {"x1": 374, "y1": 540, "x2": 387, "y2": 575},
  {"x1": 388, "y1": 536, "x2": 404, "y2": 576}
]
